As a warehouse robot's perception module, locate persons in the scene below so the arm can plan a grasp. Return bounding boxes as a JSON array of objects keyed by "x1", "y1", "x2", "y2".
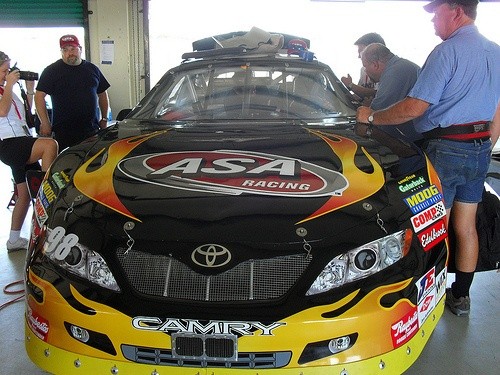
[
  {"x1": 0, "y1": 51, "x2": 59, "y2": 251},
  {"x1": 35, "y1": 35, "x2": 111, "y2": 154},
  {"x1": 342, "y1": 32, "x2": 384, "y2": 101},
  {"x1": 356, "y1": 0, "x2": 500, "y2": 317},
  {"x1": 361, "y1": 43, "x2": 424, "y2": 147}
]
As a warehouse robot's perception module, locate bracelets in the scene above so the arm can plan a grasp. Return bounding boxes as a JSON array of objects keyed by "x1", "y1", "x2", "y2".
[
  {"x1": 103, "y1": 117, "x2": 108, "y2": 121},
  {"x1": 27, "y1": 92, "x2": 34, "y2": 95},
  {"x1": 347, "y1": 83, "x2": 352, "y2": 90}
]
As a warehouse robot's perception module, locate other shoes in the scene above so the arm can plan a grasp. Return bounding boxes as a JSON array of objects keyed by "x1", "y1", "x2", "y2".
[{"x1": 446, "y1": 282, "x2": 471, "y2": 316}]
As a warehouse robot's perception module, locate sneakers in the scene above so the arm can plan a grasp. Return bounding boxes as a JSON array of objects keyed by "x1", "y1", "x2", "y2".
[{"x1": 6, "y1": 237, "x2": 29, "y2": 251}]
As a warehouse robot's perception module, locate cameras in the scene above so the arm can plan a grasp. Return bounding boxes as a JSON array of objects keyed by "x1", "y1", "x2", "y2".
[{"x1": 9, "y1": 62, "x2": 38, "y2": 81}]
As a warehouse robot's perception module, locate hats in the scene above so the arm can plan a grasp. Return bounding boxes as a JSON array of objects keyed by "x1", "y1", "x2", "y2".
[
  {"x1": 423, "y1": 0, "x2": 477, "y2": 14},
  {"x1": 60, "y1": 35, "x2": 79, "y2": 49}
]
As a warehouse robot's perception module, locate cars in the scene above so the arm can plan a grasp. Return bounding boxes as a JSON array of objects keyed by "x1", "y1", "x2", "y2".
[{"x1": 23, "y1": 29, "x2": 451, "y2": 375}]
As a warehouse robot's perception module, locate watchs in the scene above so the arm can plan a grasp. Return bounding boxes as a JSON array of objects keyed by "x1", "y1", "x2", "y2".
[{"x1": 368, "y1": 111, "x2": 375, "y2": 126}]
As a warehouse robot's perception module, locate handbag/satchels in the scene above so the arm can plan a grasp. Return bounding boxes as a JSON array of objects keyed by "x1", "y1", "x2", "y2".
[{"x1": 448, "y1": 172, "x2": 500, "y2": 273}]
